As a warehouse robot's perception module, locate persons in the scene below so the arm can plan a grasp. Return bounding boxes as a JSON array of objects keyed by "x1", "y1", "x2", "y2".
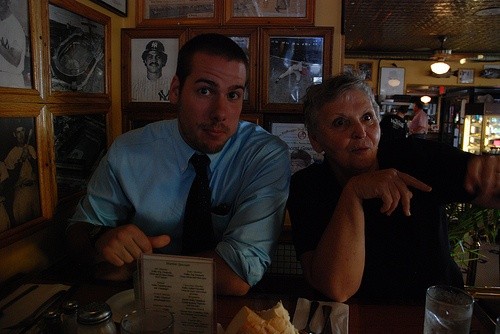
[
  {"x1": 379, "y1": 102, "x2": 428, "y2": 138},
  {"x1": 291, "y1": 150, "x2": 311, "y2": 174},
  {"x1": 287, "y1": 76, "x2": 500, "y2": 303},
  {"x1": 0, "y1": 0, "x2": 41, "y2": 230},
  {"x1": 276, "y1": 57, "x2": 308, "y2": 84},
  {"x1": 65, "y1": 33, "x2": 292, "y2": 297},
  {"x1": 133, "y1": 40, "x2": 171, "y2": 102}
]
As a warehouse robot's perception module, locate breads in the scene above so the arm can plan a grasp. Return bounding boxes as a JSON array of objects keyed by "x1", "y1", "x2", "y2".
[{"x1": 222, "y1": 300, "x2": 299, "y2": 334}]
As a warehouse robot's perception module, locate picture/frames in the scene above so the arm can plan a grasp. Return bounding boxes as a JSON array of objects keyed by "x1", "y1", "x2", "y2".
[
  {"x1": 458, "y1": 68, "x2": 474, "y2": 83},
  {"x1": 0, "y1": 0, "x2": 333, "y2": 246},
  {"x1": 380, "y1": 67, "x2": 406, "y2": 98}
]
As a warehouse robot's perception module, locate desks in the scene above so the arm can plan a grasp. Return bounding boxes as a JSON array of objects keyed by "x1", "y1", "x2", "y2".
[{"x1": 0, "y1": 273, "x2": 497, "y2": 334}]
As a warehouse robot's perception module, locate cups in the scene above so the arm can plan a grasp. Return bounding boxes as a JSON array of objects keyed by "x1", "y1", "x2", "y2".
[
  {"x1": 120, "y1": 307, "x2": 176, "y2": 334},
  {"x1": 423, "y1": 285, "x2": 474, "y2": 334}
]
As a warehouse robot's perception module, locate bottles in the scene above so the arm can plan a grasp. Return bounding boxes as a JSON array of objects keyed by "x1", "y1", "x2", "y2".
[
  {"x1": 76, "y1": 300, "x2": 117, "y2": 334},
  {"x1": 63, "y1": 301, "x2": 78, "y2": 334},
  {"x1": 42, "y1": 310, "x2": 64, "y2": 334}
]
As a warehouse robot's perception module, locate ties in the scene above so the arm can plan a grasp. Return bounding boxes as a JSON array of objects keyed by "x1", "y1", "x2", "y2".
[{"x1": 181, "y1": 152, "x2": 212, "y2": 256}]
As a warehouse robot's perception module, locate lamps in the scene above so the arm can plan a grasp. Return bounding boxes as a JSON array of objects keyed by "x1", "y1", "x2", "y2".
[{"x1": 431, "y1": 58, "x2": 450, "y2": 75}]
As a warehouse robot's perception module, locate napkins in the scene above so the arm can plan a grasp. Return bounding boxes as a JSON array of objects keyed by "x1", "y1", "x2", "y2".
[
  {"x1": 0, "y1": 281, "x2": 70, "y2": 334},
  {"x1": 292, "y1": 298, "x2": 350, "y2": 334}
]
